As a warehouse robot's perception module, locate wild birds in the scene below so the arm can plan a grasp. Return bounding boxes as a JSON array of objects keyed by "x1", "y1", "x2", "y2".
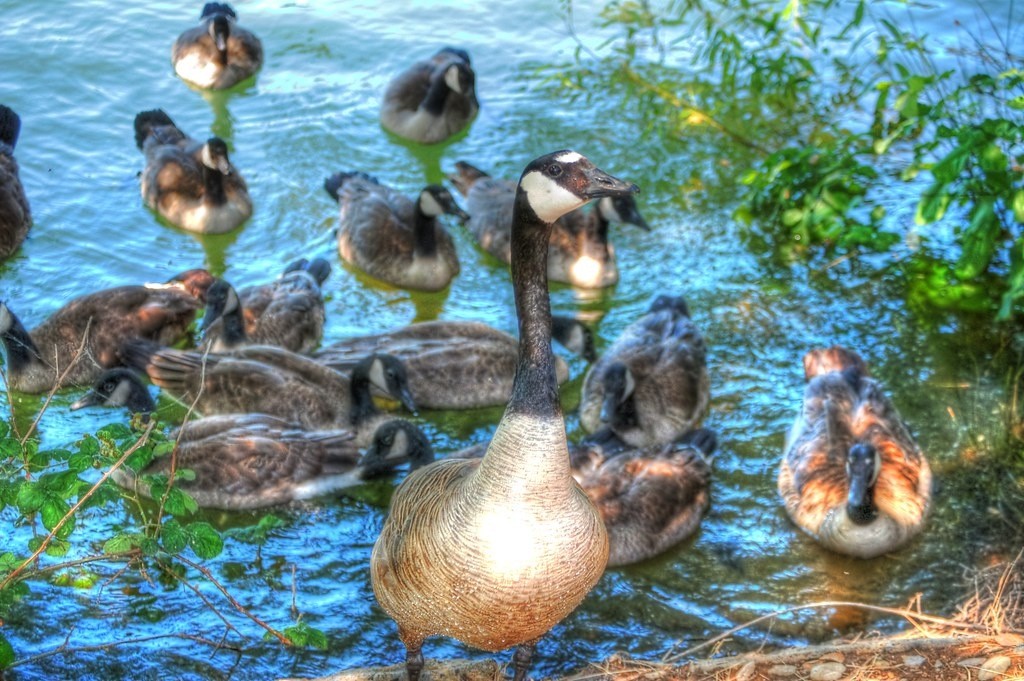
[
  {"x1": 325, "y1": 168, "x2": 470, "y2": 293},
  {"x1": 357, "y1": 420, "x2": 497, "y2": 483},
  {"x1": 172, "y1": 3, "x2": 263, "y2": 92},
  {"x1": 777, "y1": 343, "x2": 932, "y2": 559},
  {"x1": 68, "y1": 366, "x2": 373, "y2": 509},
  {"x1": 132, "y1": 107, "x2": 255, "y2": 231},
  {"x1": 569, "y1": 418, "x2": 717, "y2": 568},
  {"x1": 199, "y1": 256, "x2": 332, "y2": 347},
  {"x1": 445, "y1": 160, "x2": 652, "y2": 288},
  {"x1": 0, "y1": 103, "x2": 32, "y2": 260},
  {"x1": 0, "y1": 270, "x2": 215, "y2": 394},
  {"x1": 580, "y1": 295, "x2": 710, "y2": 444},
  {"x1": 370, "y1": 146, "x2": 641, "y2": 681},
  {"x1": 384, "y1": 46, "x2": 480, "y2": 144},
  {"x1": 200, "y1": 280, "x2": 570, "y2": 416}
]
